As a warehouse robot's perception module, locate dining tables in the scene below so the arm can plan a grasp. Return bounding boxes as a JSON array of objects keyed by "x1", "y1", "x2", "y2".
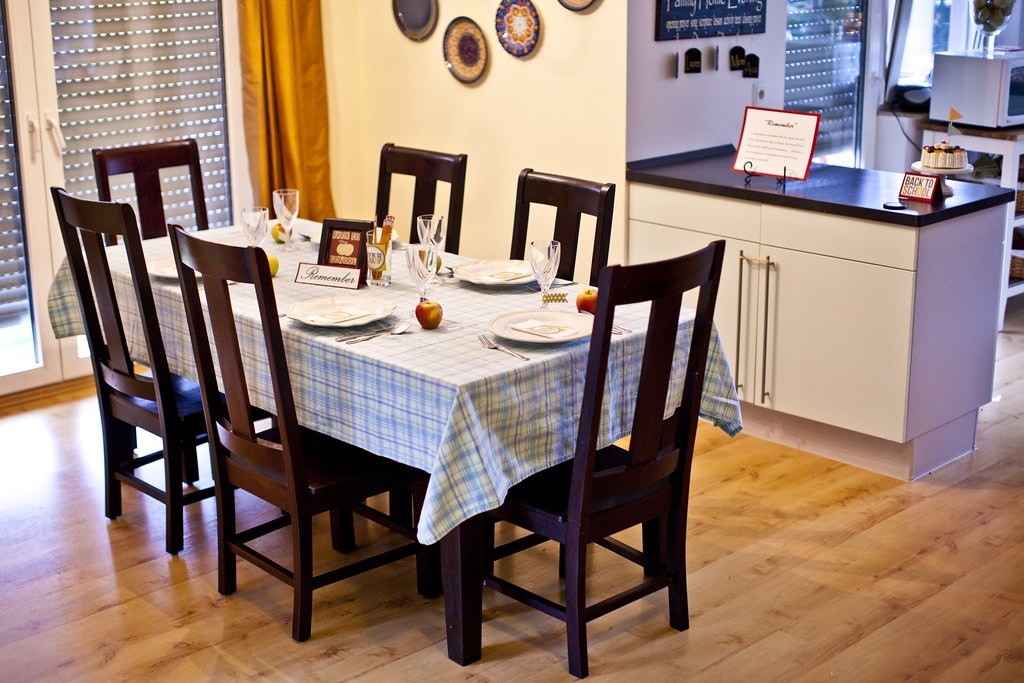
[{"x1": 47, "y1": 214, "x2": 742, "y2": 545}]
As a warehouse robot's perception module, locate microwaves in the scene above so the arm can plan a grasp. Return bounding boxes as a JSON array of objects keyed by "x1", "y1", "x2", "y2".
[{"x1": 928, "y1": 46, "x2": 1024, "y2": 130}]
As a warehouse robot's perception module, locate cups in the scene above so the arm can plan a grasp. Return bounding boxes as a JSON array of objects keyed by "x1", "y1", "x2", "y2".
[
  {"x1": 240, "y1": 207, "x2": 270, "y2": 246},
  {"x1": 367, "y1": 230, "x2": 393, "y2": 287}
]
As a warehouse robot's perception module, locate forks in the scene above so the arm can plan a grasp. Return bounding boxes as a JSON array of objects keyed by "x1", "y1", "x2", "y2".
[{"x1": 479, "y1": 335, "x2": 530, "y2": 361}]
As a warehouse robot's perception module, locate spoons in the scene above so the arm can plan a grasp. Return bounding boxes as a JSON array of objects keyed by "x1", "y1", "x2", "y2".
[{"x1": 346, "y1": 322, "x2": 412, "y2": 345}]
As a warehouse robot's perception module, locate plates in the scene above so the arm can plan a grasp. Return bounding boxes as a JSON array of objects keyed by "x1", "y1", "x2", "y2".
[
  {"x1": 556, "y1": 0, "x2": 595, "y2": 11},
  {"x1": 495, "y1": 0, "x2": 539, "y2": 57},
  {"x1": 487, "y1": 309, "x2": 594, "y2": 345},
  {"x1": 443, "y1": 16, "x2": 487, "y2": 84},
  {"x1": 285, "y1": 294, "x2": 398, "y2": 328},
  {"x1": 310, "y1": 228, "x2": 398, "y2": 248},
  {"x1": 446, "y1": 259, "x2": 536, "y2": 287},
  {"x1": 392, "y1": 0, "x2": 439, "y2": 42},
  {"x1": 143, "y1": 254, "x2": 202, "y2": 279}
]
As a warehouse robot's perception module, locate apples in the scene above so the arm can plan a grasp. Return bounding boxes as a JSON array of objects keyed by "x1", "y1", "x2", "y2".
[
  {"x1": 266, "y1": 255, "x2": 279, "y2": 276},
  {"x1": 434, "y1": 255, "x2": 441, "y2": 272},
  {"x1": 576, "y1": 289, "x2": 598, "y2": 315},
  {"x1": 415, "y1": 301, "x2": 443, "y2": 329},
  {"x1": 271, "y1": 224, "x2": 293, "y2": 243}
]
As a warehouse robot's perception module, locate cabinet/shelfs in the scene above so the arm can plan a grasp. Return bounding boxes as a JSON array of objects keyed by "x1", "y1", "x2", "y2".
[
  {"x1": 626, "y1": 148, "x2": 1015, "y2": 483},
  {"x1": 916, "y1": 119, "x2": 1024, "y2": 301}
]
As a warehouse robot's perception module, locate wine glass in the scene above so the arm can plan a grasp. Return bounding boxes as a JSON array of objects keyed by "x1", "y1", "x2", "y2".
[
  {"x1": 417, "y1": 214, "x2": 446, "y2": 284},
  {"x1": 405, "y1": 244, "x2": 438, "y2": 319},
  {"x1": 272, "y1": 189, "x2": 300, "y2": 253},
  {"x1": 529, "y1": 240, "x2": 561, "y2": 310}
]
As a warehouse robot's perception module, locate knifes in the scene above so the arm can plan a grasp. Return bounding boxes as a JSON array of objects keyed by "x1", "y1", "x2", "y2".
[{"x1": 334, "y1": 323, "x2": 395, "y2": 342}]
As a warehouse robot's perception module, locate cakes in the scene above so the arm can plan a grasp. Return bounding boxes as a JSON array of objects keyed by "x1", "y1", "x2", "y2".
[{"x1": 921, "y1": 141, "x2": 969, "y2": 169}]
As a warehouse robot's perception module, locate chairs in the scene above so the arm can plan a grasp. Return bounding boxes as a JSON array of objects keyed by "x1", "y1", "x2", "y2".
[
  {"x1": 484, "y1": 239, "x2": 727, "y2": 678},
  {"x1": 510, "y1": 168, "x2": 615, "y2": 287},
  {"x1": 167, "y1": 224, "x2": 438, "y2": 641},
  {"x1": 375, "y1": 143, "x2": 468, "y2": 255},
  {"x1": 93, "y1": 138, "x2": 209, "y2": 246},
  {"x1": 49, "y1": 184, "x2": 272, "y2": 553}
]
